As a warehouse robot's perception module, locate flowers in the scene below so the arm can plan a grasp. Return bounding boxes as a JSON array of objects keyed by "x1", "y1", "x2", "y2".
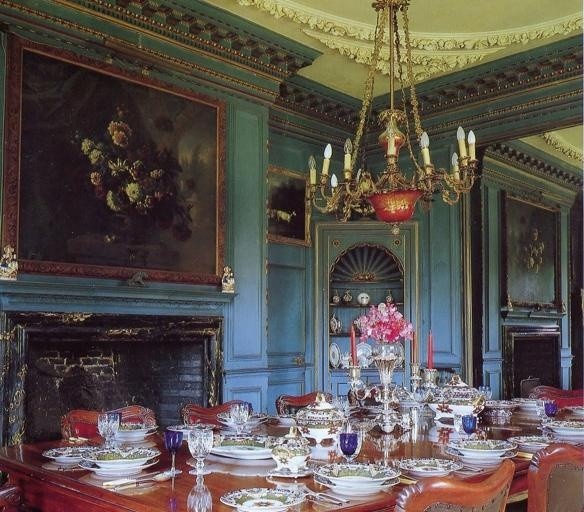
[{"x1": 352, "y1": 303, "x2": 416, "y2": 344}]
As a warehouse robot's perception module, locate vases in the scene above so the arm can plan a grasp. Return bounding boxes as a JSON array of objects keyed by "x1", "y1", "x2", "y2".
[{"x1": 366, "y1": 342, "x2": 404, "y2": 422}]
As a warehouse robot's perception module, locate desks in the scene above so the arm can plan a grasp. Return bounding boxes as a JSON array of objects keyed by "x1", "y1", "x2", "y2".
[{"x1": 2, "y1": 409, "x2": 582, "y2": 510}]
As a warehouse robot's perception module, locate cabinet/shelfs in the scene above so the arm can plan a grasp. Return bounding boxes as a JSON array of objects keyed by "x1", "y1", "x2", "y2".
[{"x1": 329, "y1": 298, "x2": 408, "y2": 341}]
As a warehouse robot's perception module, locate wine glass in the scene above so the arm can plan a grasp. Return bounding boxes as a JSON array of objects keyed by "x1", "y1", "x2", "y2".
[
  {"x1": 163, "y1": 476, "x2": 180, "y2": 510},
  {"x1": 184, "y1": 468, "x2": 213, "y2": 512}
]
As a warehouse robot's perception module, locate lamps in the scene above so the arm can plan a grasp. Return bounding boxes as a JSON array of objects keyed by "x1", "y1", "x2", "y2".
[{"x1": 305, "y1": 1, "x2": 480, "y2": 228}]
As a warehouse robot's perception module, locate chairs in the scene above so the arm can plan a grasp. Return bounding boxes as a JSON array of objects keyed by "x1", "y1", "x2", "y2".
[
  {"x1": 390, "y1": 459, "x2": 516, "y2": 512},
  {"x1": 179, "y1": 402, "x2": 255, "y2": 422},
  {"x1": 60, "y1": 407, "x2": 159, "y2": 439},
  {"x1": 526, "y1": 442, "x2": 584, "y2": 510},
  {"x1": 275, "y1": 392, "x2": 333, "y2": 415},
  {"x1": 529, "y1": 386, "x2": 584, "y2": 407}
]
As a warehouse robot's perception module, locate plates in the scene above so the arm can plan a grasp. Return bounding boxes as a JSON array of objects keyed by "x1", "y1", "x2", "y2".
[
  {"x1": 328, "y1": 339, "x2": 404, "y2": 371},
  {"x1": 38, "y1": 386, "x2": 582, "y2": 505},
  {"x1": 356, "y1": 291, "x2": 370, "y2": 305},
  {"x1": 217, "y1": 486, "x2": 307, "y2": 512}
]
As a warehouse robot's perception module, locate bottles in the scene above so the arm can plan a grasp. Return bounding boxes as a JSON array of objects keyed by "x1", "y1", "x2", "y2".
[{"x1": 361, "y1": 297, "x2": 368, "y2": 305}]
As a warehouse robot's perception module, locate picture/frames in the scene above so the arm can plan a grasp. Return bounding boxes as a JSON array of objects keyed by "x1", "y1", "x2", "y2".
[
  {"x1": 0, "y1": 28, "x2": 235, "y2": 293},
  {"x1": 266, "y1": 163, "x2": 312, "y2": 249},
  {"x1": 497, "y1": 186, "x2": 563, "y2": 314}
]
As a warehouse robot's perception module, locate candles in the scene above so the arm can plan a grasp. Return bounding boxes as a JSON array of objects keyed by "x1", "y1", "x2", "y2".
[
  {"x1": 348, "y1": 324, "x2": 359, "y2": 367},
  {"x1": 410, "y1": 329, "x2": 418, "y2": 365},
  {"x1": 426, "y1": 328, "x2": 434, "y2": 370}
]
